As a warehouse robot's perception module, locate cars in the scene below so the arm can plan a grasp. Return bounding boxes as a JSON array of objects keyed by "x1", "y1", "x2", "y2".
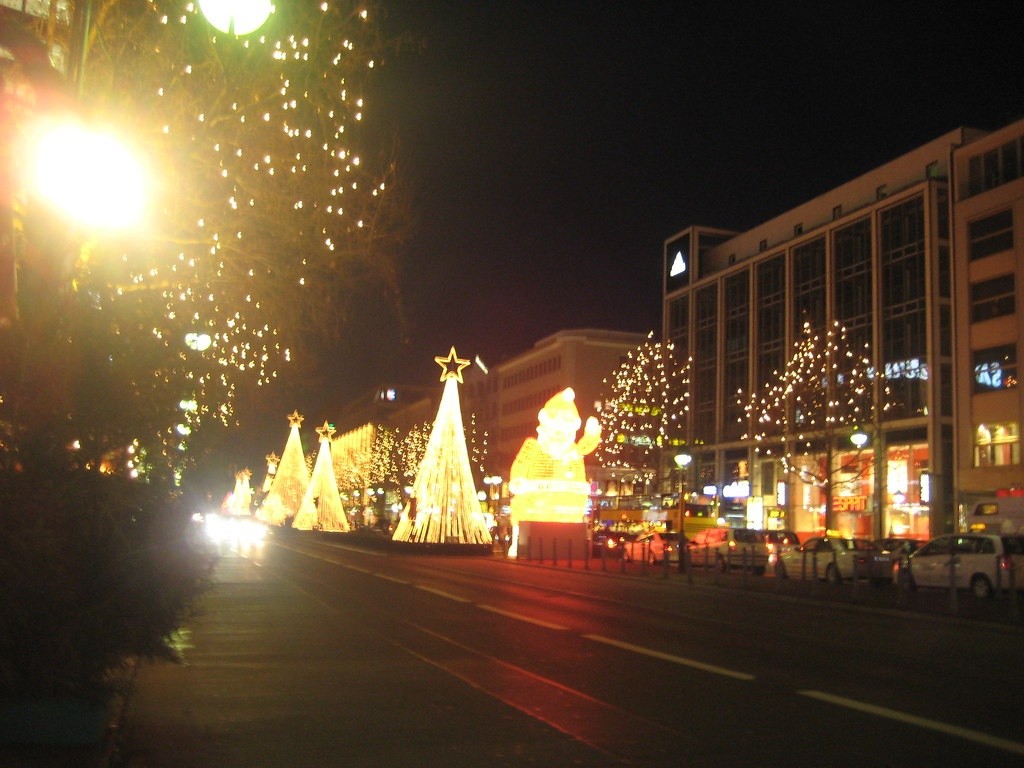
[
  {"x1": 593, "y1": 531, "x2": 632, "y2": 557},
  {"x1": 687, "y1": 527, "x2": 771, "y2": 575},
  {"x1": 620, "y1": 533, "x2": 700, "y2": 566},
  {"x1": 893, "y1": 534, "x2": 1024, "y2": 601},
  {"x1": 774, "y1": 537, "x2": 928, "y2": 583},
  {"x1": 763, "y1": 530, "x2": 801, "y2": 556}
]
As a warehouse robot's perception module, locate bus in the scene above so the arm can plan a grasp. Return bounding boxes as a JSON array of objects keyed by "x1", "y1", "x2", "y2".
[{"x1": 591, "y1": 492, "x2": 720, "y2": 540}]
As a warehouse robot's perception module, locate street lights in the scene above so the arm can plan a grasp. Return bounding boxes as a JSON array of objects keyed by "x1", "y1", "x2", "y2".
[
  {"x1": 849, "y1": 410, "x2": 883, "y2": 543},
  {"x1": 674, "y1": 444, "x2": 693, "y2": 536}
]
